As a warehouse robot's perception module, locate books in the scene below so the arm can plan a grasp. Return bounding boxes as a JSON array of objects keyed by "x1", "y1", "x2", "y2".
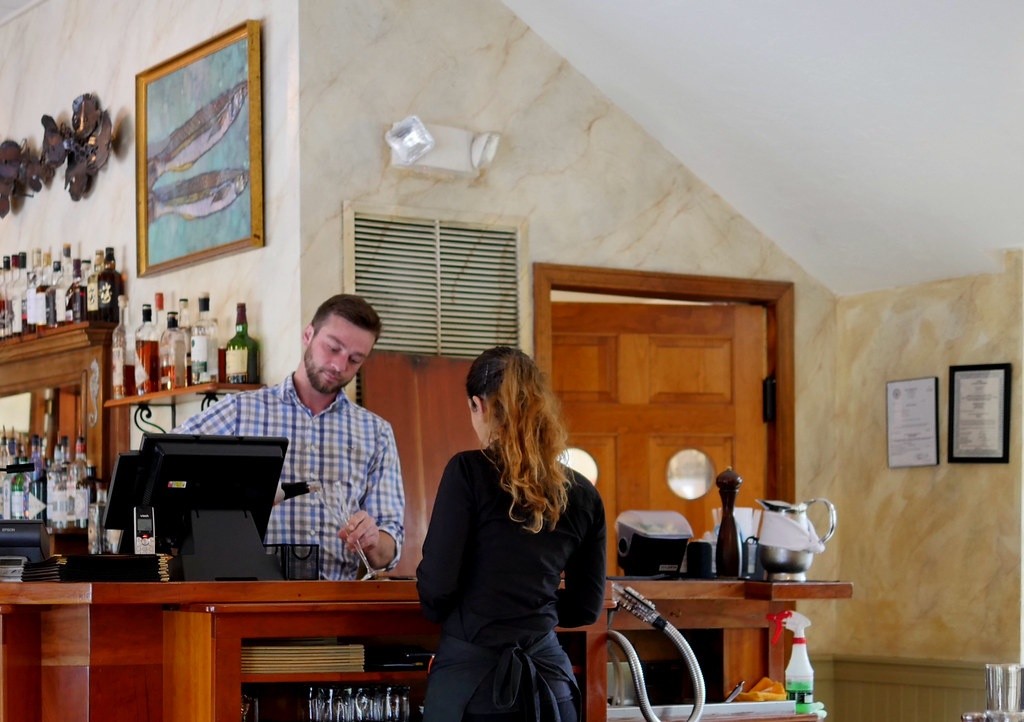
[{"x1": 21, "y1": 553, "x2": 170, "y2": 583}]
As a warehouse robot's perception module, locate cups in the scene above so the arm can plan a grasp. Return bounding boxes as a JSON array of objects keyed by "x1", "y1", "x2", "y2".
[
  {"x1": 741, "y1": 542, "x2": 763, "y2": 580},
  {"x1": 308, "y1": 686, "x2": 409, "y2": 722},
  {"x1": 985, "y1": 663, "x2": 1024, "y2": 712}
]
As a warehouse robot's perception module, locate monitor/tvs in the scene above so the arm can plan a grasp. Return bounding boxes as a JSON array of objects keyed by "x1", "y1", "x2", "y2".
[{"x1": 133, "y1": 433, "x2": 289, "y2": 582}]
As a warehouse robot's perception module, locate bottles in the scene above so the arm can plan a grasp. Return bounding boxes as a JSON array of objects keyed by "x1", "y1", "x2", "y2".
[
  {"x1": 0, "y1": 244, "x2": 124, "y2": 338},
  {"x1": 112, "y1": 296, "x2": 136, "y2": 399},
  {"x1": 160, "y1": 312, "x2": 187, "y2": 391},
  {"x1": 0, "y1": 425, "x2": 112, "y2": 555},
  {"x1": 226, "y1": 303, "x2": 260, "y2": 384},
  {"x1": 136, "y1": 304, "x2": 159, "y2": 394},
  {"x1": 153, "y1": 292, "x2": 165, "y2": 324},
  {"x1": 273, "y1": 480, "x2": 321, "y2": 505},
  {"x1": 179, "y1": 298, "x2": 191, "y2": 386},
  {"x1": 191, "y1": 292, "x2": 219, "y2": 384}
]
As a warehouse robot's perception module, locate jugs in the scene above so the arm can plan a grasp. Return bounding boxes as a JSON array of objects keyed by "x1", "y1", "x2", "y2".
[{"x1": 755, "y1": 498, "x2": 836, "y2": 580}]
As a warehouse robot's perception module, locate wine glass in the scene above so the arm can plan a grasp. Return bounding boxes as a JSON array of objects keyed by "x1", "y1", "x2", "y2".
[{"x1": 320, "y1": 482, "x2": 386, "y2": 581}]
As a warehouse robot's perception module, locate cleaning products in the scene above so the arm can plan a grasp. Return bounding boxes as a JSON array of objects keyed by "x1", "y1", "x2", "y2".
[{"x1": 767, "y1": 610, "x2": 814, "y2": 716}]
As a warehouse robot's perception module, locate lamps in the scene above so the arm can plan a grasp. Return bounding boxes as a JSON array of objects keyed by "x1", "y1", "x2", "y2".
[{"x1": 385, "y1": 116, "x2": 502, "y2": 177}]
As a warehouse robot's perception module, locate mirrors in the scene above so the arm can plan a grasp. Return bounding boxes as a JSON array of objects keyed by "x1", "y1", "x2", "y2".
[{"x1": 0, "y1": 320, "x2": 116, "y2": 488}]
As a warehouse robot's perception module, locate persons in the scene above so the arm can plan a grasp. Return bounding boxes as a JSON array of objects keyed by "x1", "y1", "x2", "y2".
[
  {"x1": 416, "y1": 347, "x2": 606, "y2": 722},
  {"x1": 169, "y1": 294, "x2": 406, "y2": 582}
]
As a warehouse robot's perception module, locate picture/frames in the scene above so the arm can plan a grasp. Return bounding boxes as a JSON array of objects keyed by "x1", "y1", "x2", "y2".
[{"x1": 131, "y1": 17, "x2": 269, "y2": 279}]
